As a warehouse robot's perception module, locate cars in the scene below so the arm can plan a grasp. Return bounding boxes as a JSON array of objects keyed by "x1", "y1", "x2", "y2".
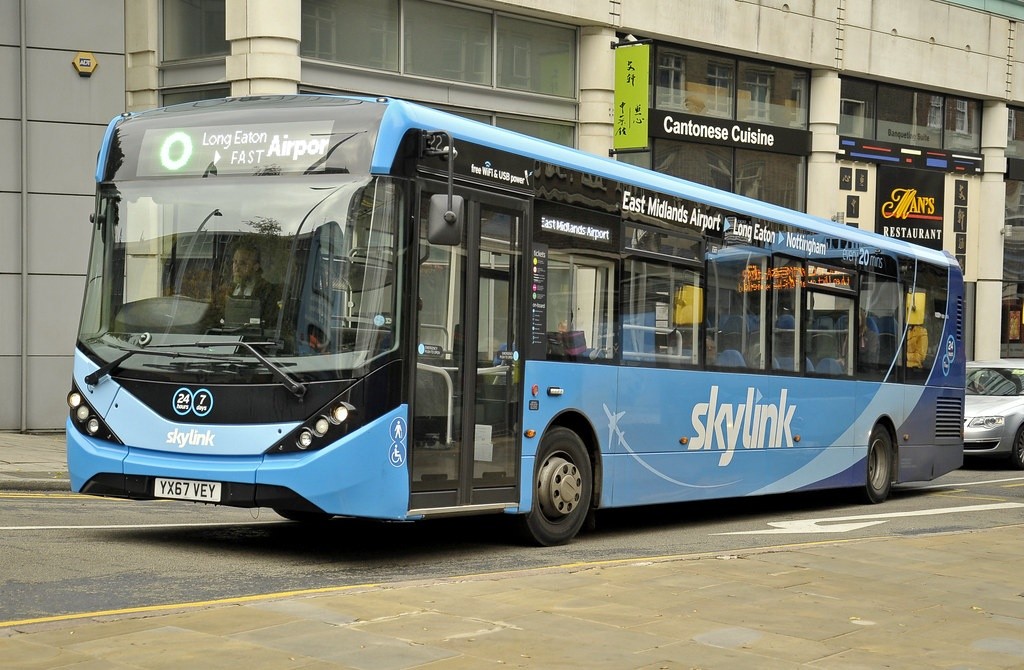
[{"x1": 963, "y1": 359, "x2": 1024, "y2": 471}]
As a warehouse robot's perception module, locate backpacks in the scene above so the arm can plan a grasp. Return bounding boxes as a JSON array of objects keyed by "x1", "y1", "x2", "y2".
[{"x1": 562, "y1": 331, "x2": 587, "y2": 356}]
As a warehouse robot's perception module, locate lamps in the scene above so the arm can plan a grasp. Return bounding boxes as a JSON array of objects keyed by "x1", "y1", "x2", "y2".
[
  {"x1": 1000, "y1": 225, "x2": 1013, "y2": 237},
  {"x1": 831, "y1": 212, "x2": 845, "y2": 224}
]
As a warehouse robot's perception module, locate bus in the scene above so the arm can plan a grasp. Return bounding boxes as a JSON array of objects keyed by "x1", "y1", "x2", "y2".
[{"x1": 65, "y1": 93, "x2": 966, "y2": 547}]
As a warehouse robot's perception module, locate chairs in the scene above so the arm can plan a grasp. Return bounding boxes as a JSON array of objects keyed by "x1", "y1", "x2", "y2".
[{"x1": 473, "y1": 307, "x2": 934, "y2": 440}]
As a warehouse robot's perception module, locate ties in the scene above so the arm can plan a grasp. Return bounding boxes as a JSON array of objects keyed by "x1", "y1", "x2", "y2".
[{"x1": 237, "y1": 285, "x2": 246, "y2": 296}]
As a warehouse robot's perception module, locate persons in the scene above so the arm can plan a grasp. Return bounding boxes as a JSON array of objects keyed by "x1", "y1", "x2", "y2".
[
  {"x1": 199, "y1": 240, "x2": 284, "y2": 341},
  {"x1": 487, "y1": 300, "x2": 931, "y2": 433}
]
